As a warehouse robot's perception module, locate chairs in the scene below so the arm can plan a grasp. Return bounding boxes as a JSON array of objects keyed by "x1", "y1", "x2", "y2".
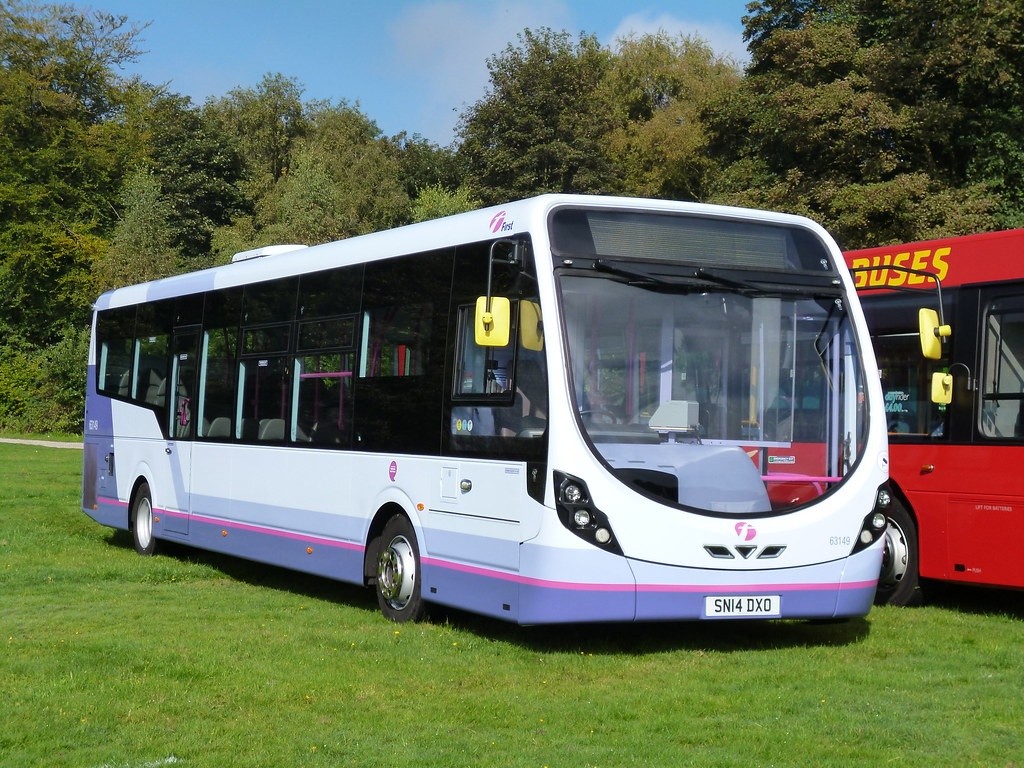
[{"x1": 118, "y1": 357, "x2": 317, "y2": 442}]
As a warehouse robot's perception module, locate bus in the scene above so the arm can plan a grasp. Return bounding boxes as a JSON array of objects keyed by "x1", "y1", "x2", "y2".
[
  {"x1": 394, "y1": 228, "x2": 1024, "y2": 609},
  {"x1": 83, "y1": 194, "x2": 953, "y2": 631}
]
